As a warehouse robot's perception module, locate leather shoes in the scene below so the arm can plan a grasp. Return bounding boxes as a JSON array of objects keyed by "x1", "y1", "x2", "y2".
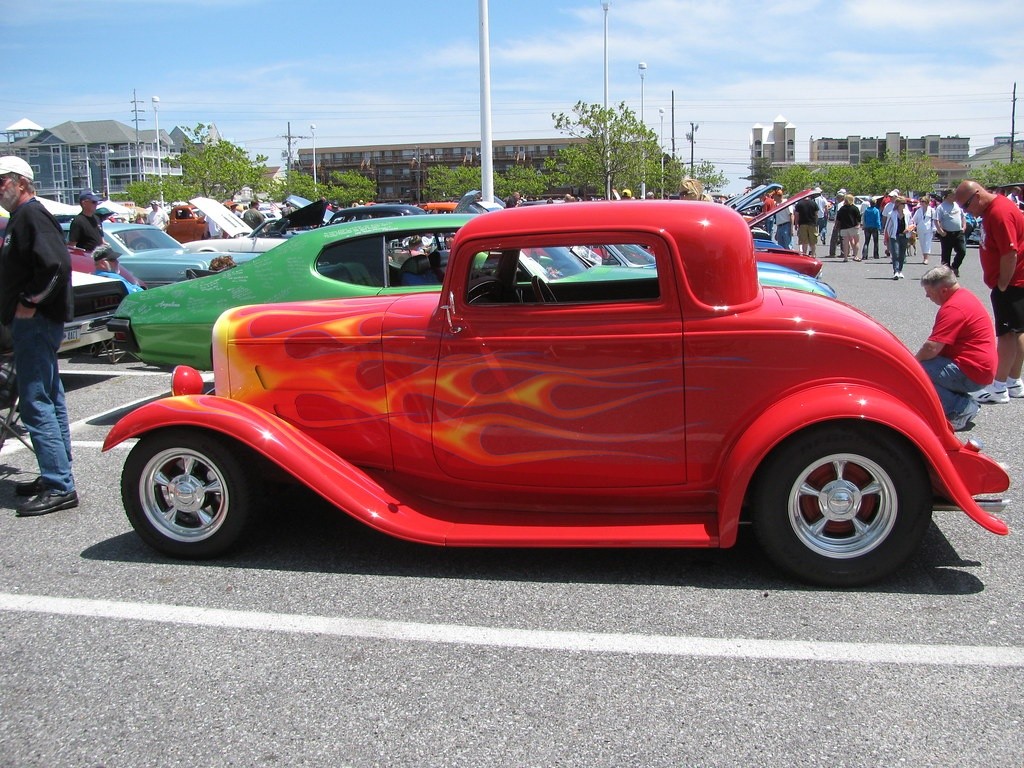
[
  {"x1": 17, "y1": 476, "x2": 49, "y2": 495},
  {"x1": 15, "y1": 488, "x2": 78, "y2": 515}
]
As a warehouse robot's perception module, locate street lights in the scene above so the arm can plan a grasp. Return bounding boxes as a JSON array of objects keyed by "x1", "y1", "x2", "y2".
[
  {"x1": 659, "y1": 108, "x2": 664, "y2": 198},
  {"x1": 637, "y1": 62, "x2": 647, "y2": 200},
  {"x1": 310, "y1": 122, "x2": 318, "y2": 194},
  {"x1": 600, "y1": 0, "x2": 612, "y2": 200},
  {"x1": 152, "y1": 96, "x2": 164, "y2": 208},
  {"x1": 105, "y1": 149, "x2": 115, "y2": 202},
  {"x1": 691, "y1": 122, "x2": 695, "y2": 180}
]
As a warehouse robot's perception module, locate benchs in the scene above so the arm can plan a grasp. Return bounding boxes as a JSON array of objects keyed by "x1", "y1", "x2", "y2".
[{"x1": 317, "y1": 255, "x2": 378, "y2": 287}]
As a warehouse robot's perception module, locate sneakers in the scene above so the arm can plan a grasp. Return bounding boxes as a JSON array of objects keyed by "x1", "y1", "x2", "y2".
[
  {"x1": 1007, "y1": 380, "x2": 1024, "y2": 398},
  {"x1": 972, "y1": 384, "x2": 1010, "y2": 404},
  {"x1": 950, "y1": 398, "x2": 982, "y2": 430}
]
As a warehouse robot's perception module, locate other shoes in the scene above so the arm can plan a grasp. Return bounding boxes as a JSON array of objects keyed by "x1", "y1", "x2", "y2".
[
  {"x1": 952, "y1": 265, "x2": 960, "y2": 277},
  {"x1": 923, "y1": 259, "x2": 928, "y2": 264},
  {"x1": 893, "y1": 272, "x2": 899, "y2": 280},
  {"x1": 899, "y1": 272, "x2": 904, "y2": 278},
  {"x1": 801, "y1": 249, "x2": 919, "y2": 265}
]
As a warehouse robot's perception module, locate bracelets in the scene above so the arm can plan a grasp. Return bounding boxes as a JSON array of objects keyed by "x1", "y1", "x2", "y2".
[{"x1": 998, "y1": 285, "x2": 1004, "y2": 291}]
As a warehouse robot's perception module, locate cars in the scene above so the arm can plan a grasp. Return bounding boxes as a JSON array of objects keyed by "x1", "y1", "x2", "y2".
[
  {"x1": 102, "y1": 198, "x2": 1011, "y2": 586},
  {"x1": 105, "y1": 184, "x2": 838, "y2": 372},
  {"x1": 0, "y1": 193, "x2": 604, "y2": 363}
]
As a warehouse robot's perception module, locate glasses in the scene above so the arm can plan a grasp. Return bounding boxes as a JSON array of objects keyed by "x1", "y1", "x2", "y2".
[
  {"x1": 961, "y1": 191, "x2": 979, "y2": 209},
  {"x1": 678, "y1": 190, "x2": 693, "y2": 196},
  {"x1": 0, "y1": 177, "x2": 8, "y2": 185}
]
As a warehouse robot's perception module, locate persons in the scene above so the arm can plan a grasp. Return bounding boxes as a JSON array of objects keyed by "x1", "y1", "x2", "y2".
[
  {"x1": 94, "y1": 196, "x2": 376, "y2": 239},
  {"x1": 397, "y1": 232, "x2": 456, "y2": 257},
  {"x1": 68, "y1": 190, "x2": 117, "y2": 355},
  {"x1": 506, "y1": 189, "x2": 669, "y2": 208},
  {"x1": 411, "y1": 199, "x2": 452, "y2": 214},
  {"x1": 914, "y1": 265, "x2": 999, "y2": 431},
  {"x1": 0, "y1": 156, "x2": 79, "y2": 516},
  {"x1": 679, "y1": 179, "x2": 753, "y2": 203},
  {"x1": 955, "y1": 180, "x2": 1024, "y2": 404},
  {"x1": 92, "y1": 244, "x2": 145, "y2": 295},
  {"x1": 884, "y1": 198, "x2": 916, "y2": 280},
  {"x1": 934, "y1": 189, "x2": 967, "y2": 277},
  {"x1": 209, "y1": 255, "x2": 236, "y2": 271},
  {"x1": 760, "y1": 186, "x2": 1024, "y2": 264}
]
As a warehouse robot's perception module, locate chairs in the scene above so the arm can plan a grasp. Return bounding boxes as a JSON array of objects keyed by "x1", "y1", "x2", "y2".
[
  {"x1": 399, "y1": 255, "x2": 437, "y2": 284},
  {"x1": 90, "y1": 314, "x2": 140, "y2": 363},
  {"x1": 429, "y1": 250, "x2": 450, "y2": 282},
  {"x1": 0, "y1": 321, "x2": 38, "y2": 456}
]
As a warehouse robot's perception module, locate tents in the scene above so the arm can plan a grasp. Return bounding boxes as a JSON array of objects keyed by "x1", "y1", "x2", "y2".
[{"x1": 0, "y1": 195, "x2": 131, "y2": 218}]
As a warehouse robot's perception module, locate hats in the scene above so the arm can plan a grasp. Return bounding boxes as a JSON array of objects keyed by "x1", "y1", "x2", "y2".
[
  {"x1": 250, "y1": 199, "x2": 258, "y2": 205},
  {"x1": 93, "y1": 245, "x2": 122, "y2": 259},
  {"x1": 895, "y1": 197, "x2": 907, "y2": 204},
  {"x1": 359, "y1": 200, "x2": 365, "y2": 205},
  {"x1": 78, "y1": 191, "x2": 102, "y2": 202},
  {"x1": 0, "y1": 155, "x2": 34, "y2": 181},
  {"x1": 286, "y1": 202, "x2": 292, "y2": 206},
  {"x1": 223, "y1": 201, "x2": 239, "y2": 208},
  {"x1": 837, "y1": 189, "x2": 846, "y2": 196},
  {"x1": 888, "y1": 191, "x2": 898, "y2": 197},
  {"x1": 622, "y1": 189, "x2": 632, "y2": 198}
]
